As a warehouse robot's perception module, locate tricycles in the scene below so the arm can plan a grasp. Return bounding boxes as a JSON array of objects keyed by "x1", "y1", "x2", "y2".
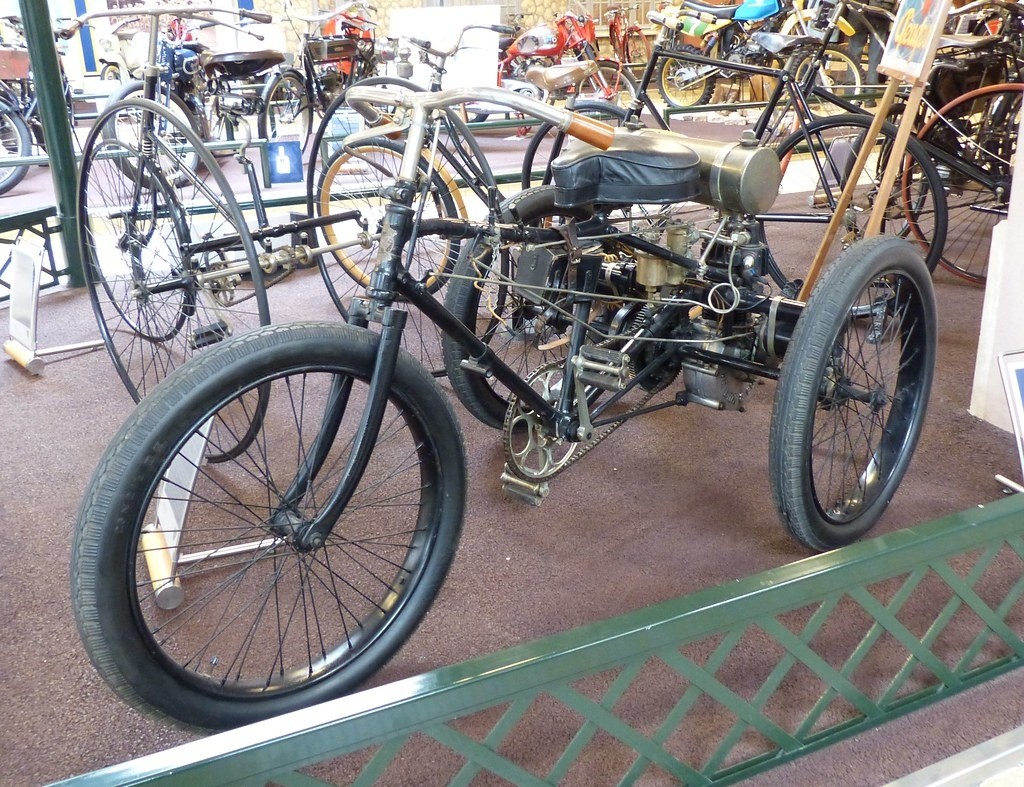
[{"x1": 1, "y1": 0, "x2": 1023, "y2": 725}]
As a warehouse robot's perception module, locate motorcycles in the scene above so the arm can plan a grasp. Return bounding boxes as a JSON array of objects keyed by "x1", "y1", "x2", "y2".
[{"x1": 448, "y1": 9, "x2": 640, "y2": 131}]
